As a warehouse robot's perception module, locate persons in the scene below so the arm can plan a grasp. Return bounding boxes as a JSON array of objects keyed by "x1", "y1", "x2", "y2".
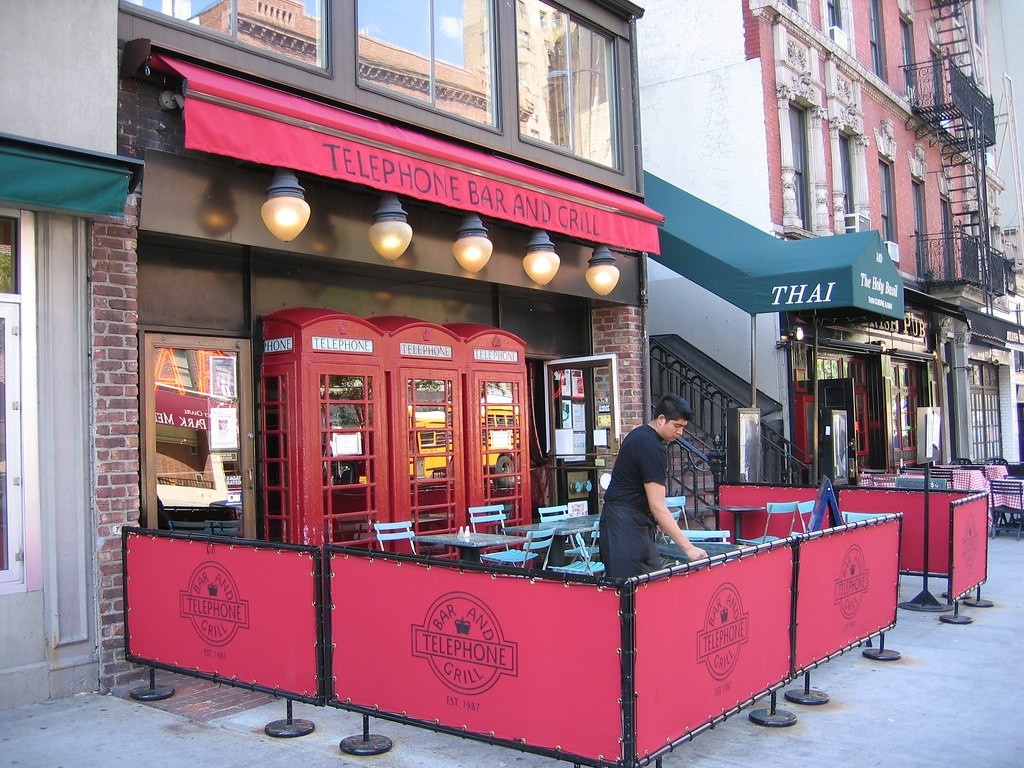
[{"x1": 599, "y1": 394, "x2": 709, "y2": 578}]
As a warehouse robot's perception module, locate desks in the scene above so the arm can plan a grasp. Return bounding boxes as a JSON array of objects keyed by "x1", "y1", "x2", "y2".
[
  {"x1": 706, "y1": 504, "x2": 765, "y2": 544},
  {"x1": 567, "y1": 513, "x2": 602, "y2": 531},
  {"x1": 658, "y1": 544, "x2": 749, "y2": 563},
  {"x1": 411, "y1": 533, "x2": 526, "y2": 561},
  {"x1": 502, "y1": 521, "x2": 594, "y2": 572},
  {"x1": 861, "y1": 465, "x2": 1024, "y2": 520}
]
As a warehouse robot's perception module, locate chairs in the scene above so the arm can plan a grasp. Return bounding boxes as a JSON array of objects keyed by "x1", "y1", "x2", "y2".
[
  {"x1": 374, "y1": 496, "x2": 892, "y2": 577},
  {"x1": 169, "y1": 520, "x2": 242, "y2": 538},
  {"x1": 900, "y1": 458, "x2": 1024, "y2": 541}
]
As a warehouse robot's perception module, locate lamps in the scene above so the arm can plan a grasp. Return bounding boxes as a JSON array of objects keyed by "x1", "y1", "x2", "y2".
[
  {"x1": 452, "y1": 212, "x2": 492, "y2": 274},
  {"x1": 585, "y1": 245, "x2": 620, "y2": 296},
  {"x1": 367, "y1": 194, "x2": 413, "y2": 260},
  {"x1": 261, "y1": 166, "x2": 310, "y2": 244},
  {"x1": 522, "y1": 227, "x2": 561, "y2": 285}
]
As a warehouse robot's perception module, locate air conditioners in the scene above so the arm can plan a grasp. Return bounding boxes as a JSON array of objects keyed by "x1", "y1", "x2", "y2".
[
  {"x1": 845, "y1": 212, "x2": 871, "y2": 233},
  {"x1": 829, "y1": 26, "x2": 847, "y2": 52},
  {"x1": 884, "y1": 241, "x2": 900, "y2": 263}
]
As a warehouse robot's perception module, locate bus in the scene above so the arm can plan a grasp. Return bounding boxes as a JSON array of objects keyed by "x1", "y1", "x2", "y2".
[{"x1": 408, "y1": 406, "x2": 520, "y2": 487}]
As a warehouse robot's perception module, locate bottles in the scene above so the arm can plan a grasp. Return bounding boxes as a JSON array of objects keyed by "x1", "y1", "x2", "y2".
[{"x1": 458, "y1": 526, "x2": 470, "y2": 539}]
